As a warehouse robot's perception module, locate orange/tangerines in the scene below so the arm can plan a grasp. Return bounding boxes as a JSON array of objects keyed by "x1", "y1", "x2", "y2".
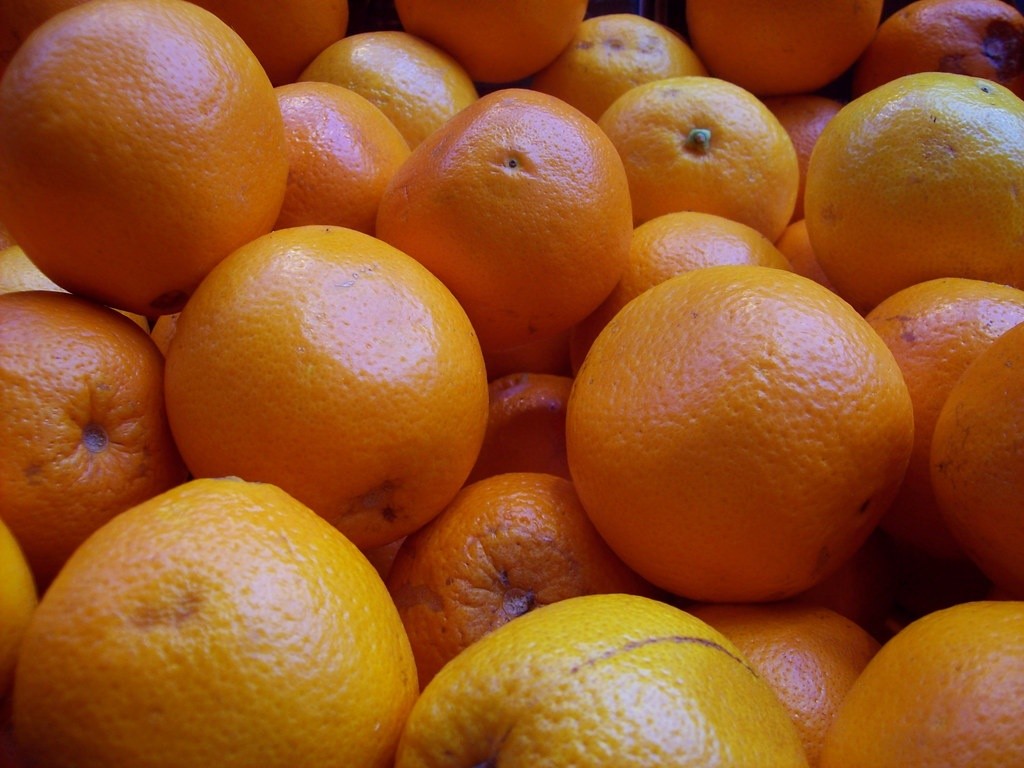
[{"x1": 0, "y1": 0, "x2": 1024, "y2": 768}]
